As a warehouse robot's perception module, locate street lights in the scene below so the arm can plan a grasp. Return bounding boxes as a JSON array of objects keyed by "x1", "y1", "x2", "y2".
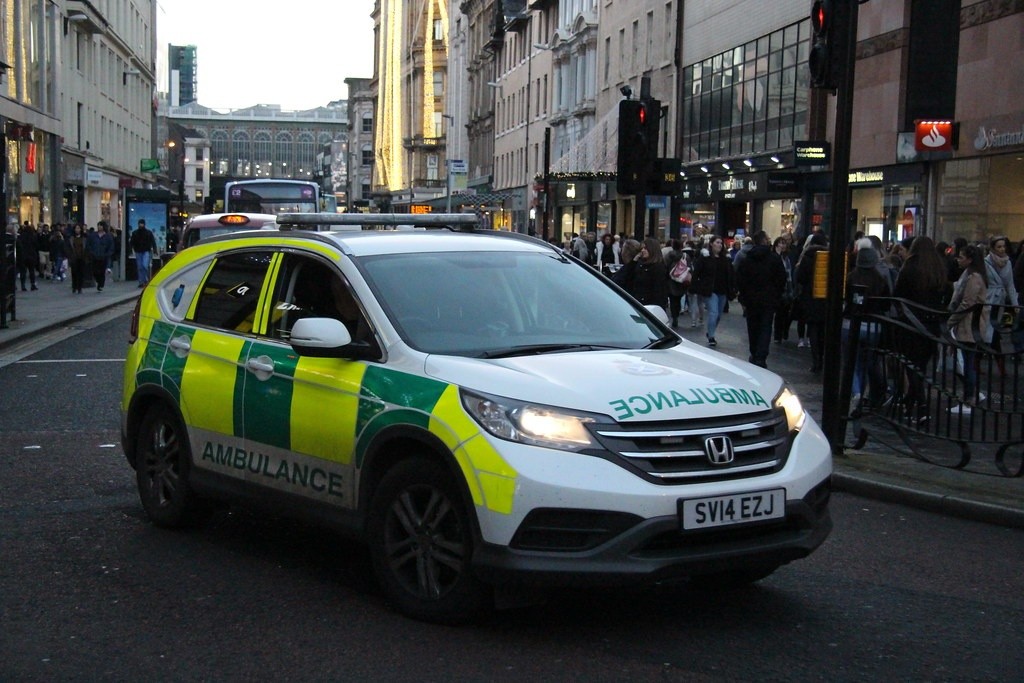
[{"x1": 346, "y1": 119, "x2": 353, "y2": 210}]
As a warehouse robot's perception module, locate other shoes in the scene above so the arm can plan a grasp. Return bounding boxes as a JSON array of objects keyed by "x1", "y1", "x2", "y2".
[
  {"x1": 672, "y1": 322, "x2": 680, "y2": 328},
  {"x1": 138, "y1": 282, "x2": 150, "y2": 288},
  {"x1": 96, "y1": 286, "x2": 103, "y2": 294},
  {"x1": 71, "y1": 288, "x2": 77, "y2": 294},
  {"x1": 78, "y1": 288, "x2": 82, "y2": 295},
  {"x1": 775, "y1": 340, "x2": 782, "y2": 347}
]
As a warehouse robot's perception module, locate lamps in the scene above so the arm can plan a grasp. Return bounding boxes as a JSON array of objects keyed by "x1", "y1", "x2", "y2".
[
  {"x1": 122, "y1": 68, "x2": 140, "y2": 86},
  {"x1": 621, "y1": 85, "x2": 633, "y2": 100},
  {"x1": 532, "y1": 43, "x2": 548, "y2": 51},
  {"x1": 442, "y1": 114, "x2": 455, "y2": 118},
  {"x1": 487, "y1": 82, "x2": 504, "y2": 89},
  {"x1": 63, "y1": 13, "x2": 87, "y2": 35}
]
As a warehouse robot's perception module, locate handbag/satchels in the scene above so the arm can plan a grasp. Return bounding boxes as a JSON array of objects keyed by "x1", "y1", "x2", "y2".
[{"x1": 670, "y1": 252, "x2": 692, "y2": 284}]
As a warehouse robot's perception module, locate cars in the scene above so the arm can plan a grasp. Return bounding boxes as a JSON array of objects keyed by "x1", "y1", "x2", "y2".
[
  {"x1": 176, "y1": 213, "x2": 284, "y2": 253},
  {"x1": 337, "y1": 202, "x2": 347, "y2": 213}
]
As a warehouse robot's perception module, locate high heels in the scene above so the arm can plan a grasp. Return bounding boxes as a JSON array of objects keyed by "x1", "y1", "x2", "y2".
[
  {"x1": 21, "y1": 285, "x2": 28, "y2": 293},
  {"x1": 29, "y1": 284, "x2": 37, "y2": 291}
]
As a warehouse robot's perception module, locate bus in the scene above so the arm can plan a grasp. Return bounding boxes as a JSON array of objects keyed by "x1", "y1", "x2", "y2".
[
  {"x1": 223, "y1": 179, "x2": 326, "y2": 222},
  {"x1": 352, "y1": 199, "x2": 370, "y2": 211}
]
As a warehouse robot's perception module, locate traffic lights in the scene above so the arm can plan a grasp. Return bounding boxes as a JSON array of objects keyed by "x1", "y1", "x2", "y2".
[{"x1": 635, "y1": 98, "x2": 652, "y2": 150}]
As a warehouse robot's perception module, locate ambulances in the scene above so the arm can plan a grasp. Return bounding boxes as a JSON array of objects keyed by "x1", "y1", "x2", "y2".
[{"x1": 121, "y1": 212, "x2": 833, "y2": 626}]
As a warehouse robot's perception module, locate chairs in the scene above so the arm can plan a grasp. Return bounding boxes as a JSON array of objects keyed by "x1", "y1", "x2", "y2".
[
  {"x1": 299, "y1": 278, "x2": 333, "y2": 315},
  {"x1": 409, "y1": 263, "x2": 466, "y2": 323}
]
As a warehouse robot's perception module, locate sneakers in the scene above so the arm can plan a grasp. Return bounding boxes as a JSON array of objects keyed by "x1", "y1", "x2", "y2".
[
  {"x1": 847, "y1": 391, "x2": 867, "y2": 419},
  {"x1": 976, "y1": 392, "x2": 985, "y2": 404},
  {"x1": 948, "y1": 404, "x2": 972, "y2": 414},
  {"x1": 808, "y1": 340, "x2": 811, "y2": 348},
  {"x1": 699, "y1": 321, "x2": 704, "y2": 327},
  {"x1": 797, "y1": 339, "x2": 805, "y2": 349},
  {"x1": 706, "y1": 330, "x2": 718, "y2": 349},
  {"x1": 691, "y1": 321, "x2": 696, "y2": 327}
]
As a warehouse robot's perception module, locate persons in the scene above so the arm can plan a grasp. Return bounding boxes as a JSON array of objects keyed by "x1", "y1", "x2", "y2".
[
  {"x1": 695, "y1": 235, "x2": 732, "y2": 345},
  {"x1": 611, "y1": 238, "x2": 641, "y2": 292},
  {"x1": 633, "y1": 238, "x2": 668, "y2": 303},
  {"x1": 87, "y1": 221, "x2": 115, "y2": 294},
  {"x1": 131, "y1": 218, "x2": 157, "y2": 287},
  {"x1": 732, "y1": 230, "x2": 788, "y2": 368},
  {"x1": 947, "y1": 246, "x2": 989, "y2": 415},
  {"x1": 548, "y1": 221, "x2": 1024, "y2": 417},
  {"x1": 895, "y1": 235, "x2": 941, "y2": 412},
  {"x1": 846, "y1": 246, "x2": 892, "y2": 414},
  {"x1": 0, "y1": 219, "x2": 188, "y2": 329},
  {"x1": 63, "y1": 225, "x2": 86, "y2": 293}
]
